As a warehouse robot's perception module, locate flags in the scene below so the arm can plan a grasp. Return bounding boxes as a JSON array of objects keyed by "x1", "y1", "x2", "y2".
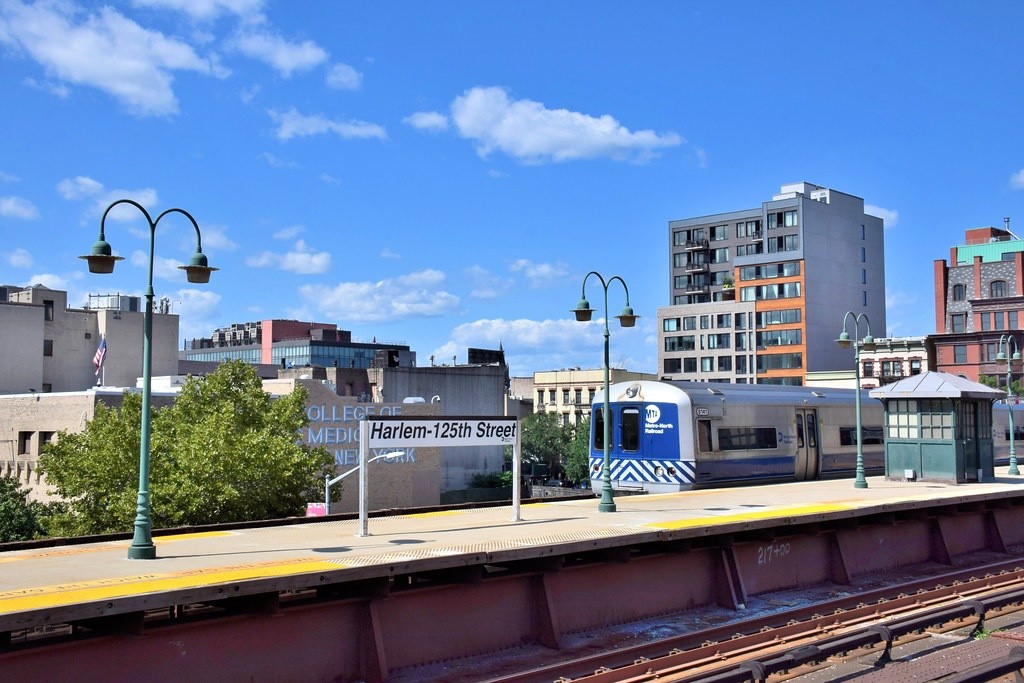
[{"x1": 93, "y1": 338, "x2": 107, "y2": 375}]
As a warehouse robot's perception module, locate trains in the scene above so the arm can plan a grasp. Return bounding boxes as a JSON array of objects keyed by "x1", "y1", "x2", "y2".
[{"x1": 588, "y1": 380, "x2": 889, "y2": 494}]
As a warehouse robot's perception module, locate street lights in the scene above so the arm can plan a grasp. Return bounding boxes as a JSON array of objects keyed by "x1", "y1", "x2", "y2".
[
  {"x1": 76, "y1": 199, "x2": 219, "y2": 559},
  {"x1": 995, "y1": 335, "x2": 1023, "y2": 474},
  {"x1": 324, "y1": 452, "x2": 405, "y2": 516},
  {"x1": 833, "y1": 311, "x2": 878, "y2": 491},
  {"x1": 569, "y1": 271, "x2": 642, "y2": 514}
]
